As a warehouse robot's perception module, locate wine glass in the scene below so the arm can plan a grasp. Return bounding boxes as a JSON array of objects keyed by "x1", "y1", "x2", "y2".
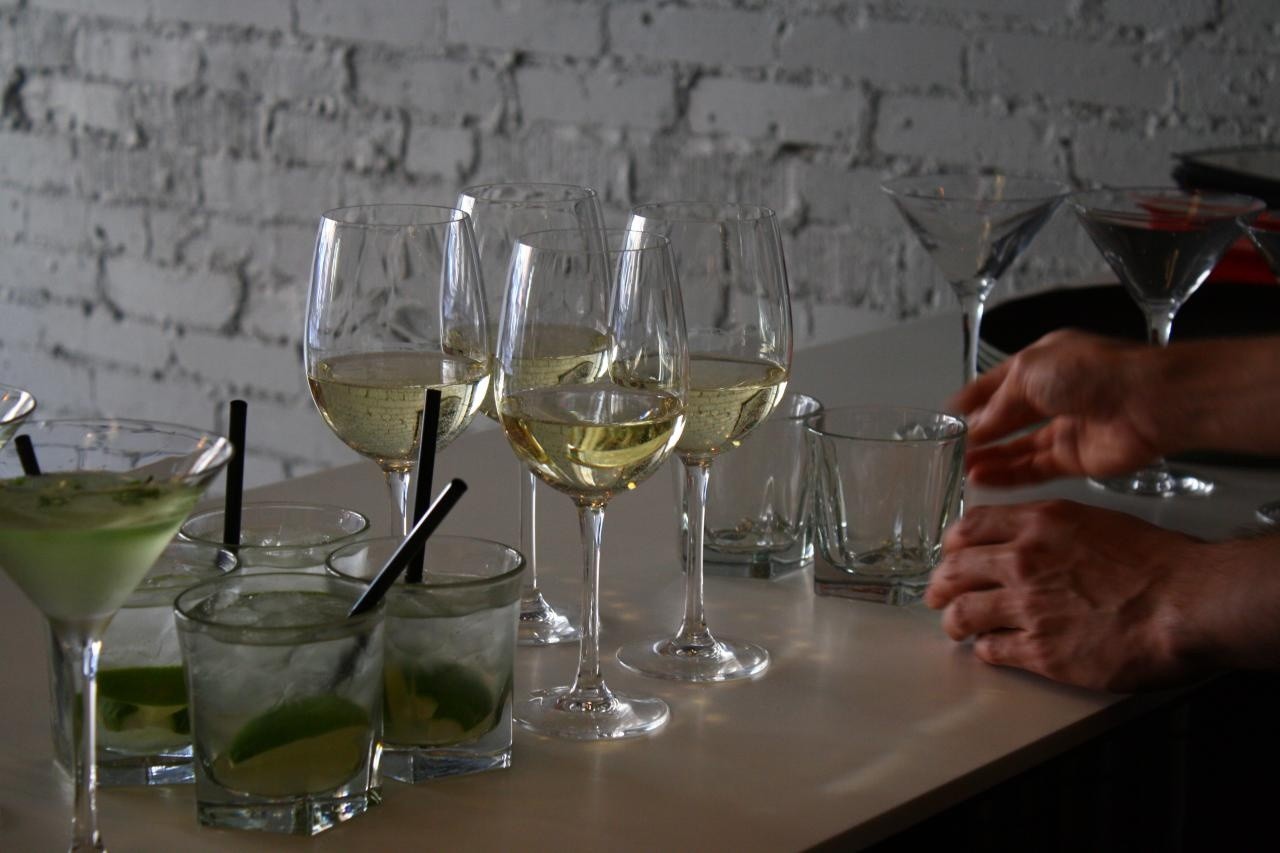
[
  {"x1": 435, "y1": 179, "x2": 616, "y2": 649},
  {"x1": 303, "y1": 200, "x2": 494, "y2": 539},
  {"x1": 490, "y1": 225, "x2": 693, "y2": 746},
  {"x1": 1062, "y1": 185, "x2": 1268, "y2": 502},
  {"x1": 607, "y1": 199, "x2": 795, "y2": 686},
  {"x1": 875, "y1": 172, "x2": 1072, "y2": 387},
  {"x1": 0, "y1": 418, "x2": 237, "y2": 853},
  {"x1": 1234, "y1": 203, "x2": 1280, "y2": 528}
]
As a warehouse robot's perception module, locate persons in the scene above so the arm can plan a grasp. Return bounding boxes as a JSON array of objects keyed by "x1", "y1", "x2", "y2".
[{"x1": 922, "y1": 329, "x2": 1279, "y2": 694}]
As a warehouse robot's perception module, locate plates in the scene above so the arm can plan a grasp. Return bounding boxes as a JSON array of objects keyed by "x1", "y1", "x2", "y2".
[{"x1": 977, "y1": 280, "x2": 1280, "y2": 471}]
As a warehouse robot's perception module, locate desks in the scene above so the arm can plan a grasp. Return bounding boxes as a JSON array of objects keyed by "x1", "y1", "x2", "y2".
[{"x1": 0, "y1": 300, "x2": 1280, "y2": 853}]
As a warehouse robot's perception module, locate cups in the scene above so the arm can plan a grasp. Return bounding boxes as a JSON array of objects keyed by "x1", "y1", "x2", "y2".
[
  {"x1": 804, "y1": 404, "x2": 968, "y2": 608},
  {"x1": 669, "y1": 389, "x2": 825, "y2": 581},
  {"x1": 174, "y1": 571, "x2": 390, "y2": 839},
  {"x1": 47, "y1": 537, "x2": 243, "y2": 790},
  {"x1": 322, "y1": 535, "x2": 528, "y2": 785},
  {"x1": 176, "y1": 501, "x2": 369, "y2": 575}
]
